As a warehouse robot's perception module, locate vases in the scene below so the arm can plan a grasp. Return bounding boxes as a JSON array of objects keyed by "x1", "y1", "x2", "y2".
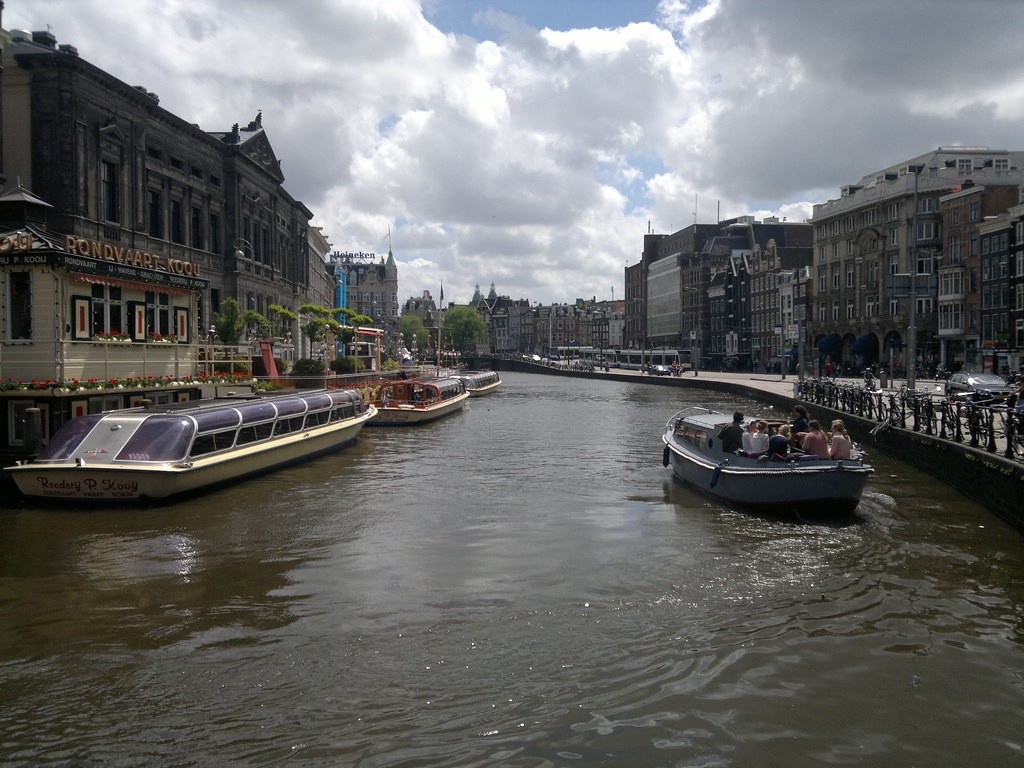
[
  {"x1": 146, "y1": 338, "x2": 164, "y2": 344},
  {"x1": 54, "y1": 382, "x2": 179, "y2": 397},
  {"x1": 120, "y1": 338, "x2": 132, "y2": 341},
  {"x1": 164, "y1": 338, "x2": 178, "y2": 343},
  {"x1": 0, "y1": 387, "x2": 53, "y2": 397},
  {"x1": 92, "y1": 334, "x2": 111, "y2": 341},
  {"x1": 178, "y1": 381, "x2": 219, "y2": 389},
  {"x1": 112, "y1": 337, "x2": 122, "y2": 341}
]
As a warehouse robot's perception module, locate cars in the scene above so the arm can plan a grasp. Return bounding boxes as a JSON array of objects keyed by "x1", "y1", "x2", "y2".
[
  {"x1": 652, "y1": 364, "x2": 672, "y2": 377},
  {"x1": 945, "y1": 371, "x2": 1015, "y2": 407}
]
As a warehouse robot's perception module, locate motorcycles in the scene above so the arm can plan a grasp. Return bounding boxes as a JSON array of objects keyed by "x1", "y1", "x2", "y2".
[{"x1": 935, "y1": 369, "x2": 951, "y2": 382}]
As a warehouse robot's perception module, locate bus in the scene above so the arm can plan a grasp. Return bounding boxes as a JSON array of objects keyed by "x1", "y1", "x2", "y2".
[
  {"x1": 620, "y1": 350, "x2": 681, "y2": 372},
  {"x1": 549, "y1": 347, "x2": 593, "y2": 363},
  {"x1": 578, "y1": 347, "x2": 621, "y2": 368}
]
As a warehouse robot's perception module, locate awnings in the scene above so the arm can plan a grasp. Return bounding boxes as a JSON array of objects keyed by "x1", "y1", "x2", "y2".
[{"x1": 784, "y1": 349, "x2": 798, "y2": 357}]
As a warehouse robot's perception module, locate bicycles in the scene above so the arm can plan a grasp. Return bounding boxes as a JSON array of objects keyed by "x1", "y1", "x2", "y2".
[{"x1": 798, "y1": 378, "x2": 1024, "y2": 462}]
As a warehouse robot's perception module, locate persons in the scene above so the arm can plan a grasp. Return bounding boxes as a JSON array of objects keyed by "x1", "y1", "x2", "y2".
[
  {"x1": 606, "y1": 360, "x2": 685, "y2": 377},
  {"x1": 823, "y1": 355, "x2": 953, "y2": 392},
  {"x1": 717, "y1": 409, "x2": 745, "y2": 454},
  {"x1": 740, "y1": 419, "x2": 756, "y2": 453},
  {"x1": 829, "y1": 419, "x2": 851, "y2": 459},
  {"x1": 767, "y1": 424, "x2": 790, "y2": 457},
  {"x1": 751, "y1": 421, "x2": 769, "y2": 454},
  {"x1": 796, "y1": 420, "x2": 831, "y2": 459},
  {"x1": 793, "y1": 405, "x2": 810, "y2": 436}
]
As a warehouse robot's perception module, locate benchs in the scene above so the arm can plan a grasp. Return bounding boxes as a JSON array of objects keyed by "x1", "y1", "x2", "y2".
[{"x1": 724, "y1": 449, "x2": 862, "y2": 467}]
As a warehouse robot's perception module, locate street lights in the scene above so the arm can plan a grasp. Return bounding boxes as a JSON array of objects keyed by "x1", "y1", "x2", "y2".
[
  {"x1": 324, "y1": 323, "x2": 330, "y2": 370},
  {"x1": 399, "y1": 333, "x2": 403, "y2": 365},
  {"x1": 208, "y1": 324, "x2": 216, "y2": 373},
  {"x1": 685, "y1": 286, "x2": 699, "y2": 377},
  {"x1": 634, "y1": 298, "x2": 646, "y2": 374},
  {"x1": 413, "y1": 334, "x2": 417, "y2": 369}
]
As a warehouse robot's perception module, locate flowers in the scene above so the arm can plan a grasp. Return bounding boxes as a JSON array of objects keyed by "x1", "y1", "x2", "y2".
[
  {"x1": 157, "y1": 374, "x2": 173, "y2": 386},
  {"x1": 181, "y1": 376, "x2": 193, "y2": 384},
  {"x1": 198, "y1": 371, "x2": 254, "y2": 384},
  {"x1": 164, "y1": 334, "x2": 179, "y2": 343},
  {"x1": 101, "y1": 377, "x2": 119, "y2": 389},
  {"x1": 120, "y1": 332, "x2": 131, "y2": 342},
  {"x1": 97, "y1": 331, "x2": 111, "y2": 339},
  {"x1": 0, "y1": 376, "x2": 24, "y2": 391},
  {"x1": 27, "y1": 379, "x2": 57, "y2": 390},
  {"x1": 137, "y1": 376, "x2": 156, "y2": 388},
  {"x1": 54, "y1": 377, "x2": 82, "y2": 391},
  {"x1": 111, "y1": 331, "x2": 120, "y2": 341},
  {"x1": 121, "y1": 376, "x2": 139, "y2": 388},
  {"x1": 83, "y1": 378, "x2": 100, "y2": 388},
  {"x1": 146, "y1": 332, "x2": 163, "y2": 341}
]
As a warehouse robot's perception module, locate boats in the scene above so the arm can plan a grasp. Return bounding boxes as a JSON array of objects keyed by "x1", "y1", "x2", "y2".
[
  {"x1": 449, "y1": 370, "x2": 502, "y2": 398},
  {"x1": 3, "y1": 389, "x2": 379, "y2": 508},
  {"x1": 364, "y1": 376, "x2": 470, "y2": 427},
  {"x1": 661, "y1": 407, "x2": 875, "y2": 518}
]
